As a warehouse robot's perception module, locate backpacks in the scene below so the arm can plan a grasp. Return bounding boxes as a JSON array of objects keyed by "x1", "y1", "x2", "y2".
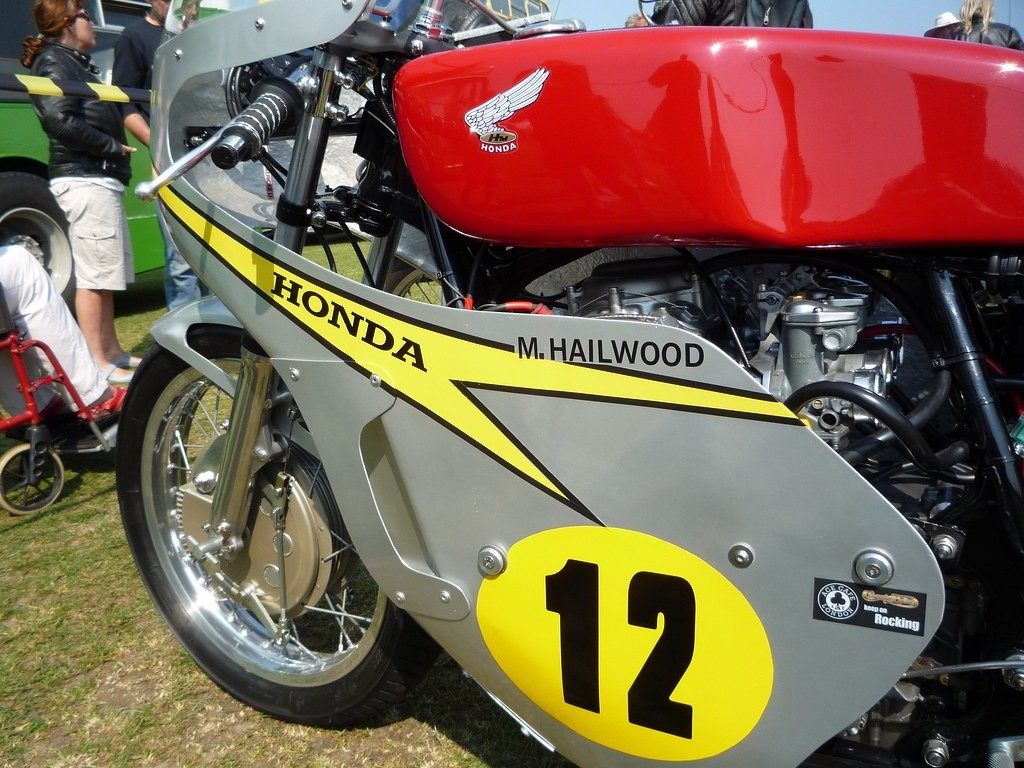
[{"x1": 739, "y1": 0, "x2": 814, "y2": 28}]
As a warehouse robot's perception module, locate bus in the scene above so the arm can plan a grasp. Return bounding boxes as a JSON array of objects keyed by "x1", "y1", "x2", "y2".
[{"x1": 0, "y1": 0, "x2": 230, "y2": 311}]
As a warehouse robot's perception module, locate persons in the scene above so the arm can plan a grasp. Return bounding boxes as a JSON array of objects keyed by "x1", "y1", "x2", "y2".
[
  {"x1": 173, "y1": 0, "x2": 202, "y2": 33},
  {"x1": 21, "y1": 1, "x2": 142, "y2": 386},
  {"x1": 112, "y1": 0, "x2": 211, "y2": 309},
  {"x1": 924, "y1": 0, "x2": 1024, "y2": 51},
  {"x1": 0, "y1": 245, "x2": 126, "y2": 428}
]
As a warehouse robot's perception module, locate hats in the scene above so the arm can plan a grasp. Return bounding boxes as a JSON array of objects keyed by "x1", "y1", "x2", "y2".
[{"x1": 924, "y1": 12, "x2": 962, "y2": 38}]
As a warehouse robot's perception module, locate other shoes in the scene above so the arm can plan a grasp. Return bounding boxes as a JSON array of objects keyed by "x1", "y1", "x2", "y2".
[
  {"x1": 108, "y1": 351, "x2": 144, "y2": 368},
  {"x1": 101, "y1": 363, "x2": 136, "y2": 383},
  {"x1": 39, "y1": 394, "x2": 74, "y2": 415},
  {"x1": 76, "y1": 387, "x2": 128, "y2": 424}
]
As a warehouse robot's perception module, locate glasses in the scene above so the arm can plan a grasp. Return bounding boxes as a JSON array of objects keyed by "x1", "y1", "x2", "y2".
[{"x1": 66, "y1": 9, "x2": 91, "y2": 23}]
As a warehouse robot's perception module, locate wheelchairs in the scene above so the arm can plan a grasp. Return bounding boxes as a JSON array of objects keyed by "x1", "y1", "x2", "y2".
[{"x1": 0, "y1": 284, "x2": 121, "y2": 516}]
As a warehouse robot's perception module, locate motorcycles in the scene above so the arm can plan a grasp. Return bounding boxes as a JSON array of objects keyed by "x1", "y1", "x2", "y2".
[{"x1": 115, "y1": 0, "x2": 1024, "y2": 767}]
